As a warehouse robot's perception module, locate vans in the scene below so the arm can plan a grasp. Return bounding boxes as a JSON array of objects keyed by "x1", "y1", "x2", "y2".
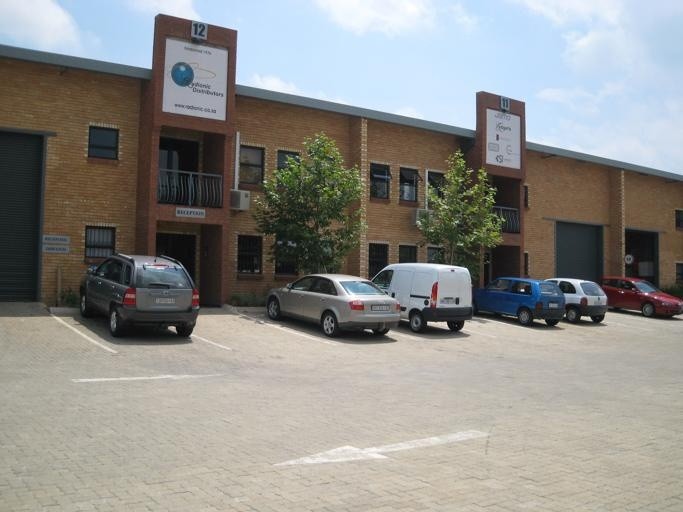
[{"x1": 369, "y1": 262, "x2": 474, "y2": 334}]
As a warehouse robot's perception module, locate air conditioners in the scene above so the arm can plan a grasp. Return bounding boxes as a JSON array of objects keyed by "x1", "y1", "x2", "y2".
[
  {"x1": 230, "y1": 188, "x2": 250, "y2": 210},
  {"x1": 412, "y1": 209, "x2": 433, "y2": 226}
]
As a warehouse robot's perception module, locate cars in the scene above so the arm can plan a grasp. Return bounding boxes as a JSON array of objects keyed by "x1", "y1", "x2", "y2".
[
  {"x1": 598, "y1": 275, "x2": 682, "y2": 318},
  {"x1": 265, "y1": 272, "x2": 402, "y2": 337}
]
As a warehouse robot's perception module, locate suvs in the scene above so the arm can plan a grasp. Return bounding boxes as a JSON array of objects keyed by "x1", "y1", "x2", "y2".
[
  {"x1": 543, "y1": 277, "x2": 608, "y2": 324},
  {"x1": 470, "y1": 276, "x2": 566, "y2": 326},
  {"x1": 81, "y1": 253, "x2": 201, "y2": 340}
]
